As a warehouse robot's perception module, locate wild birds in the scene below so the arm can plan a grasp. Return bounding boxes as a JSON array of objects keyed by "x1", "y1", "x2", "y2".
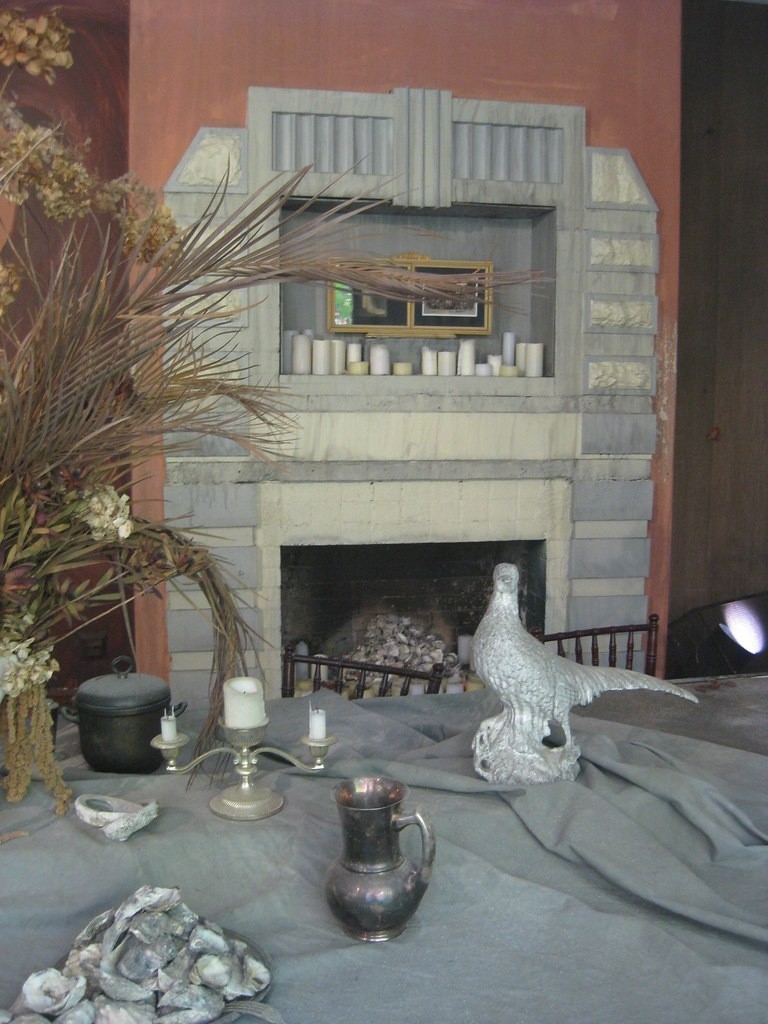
[{"x1": 468, "y1": 563, "x2": 698, "y2": 746}]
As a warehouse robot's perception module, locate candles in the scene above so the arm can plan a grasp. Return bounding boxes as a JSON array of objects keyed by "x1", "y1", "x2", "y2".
[
  {"x1": 309, "y1": 701, "x2": 326, "y2": 740},
  {"x1": 280, "y1": 329, "x2": 544, "y2": 378},
  {"x1": 160, "y1": 705, "x2": 178, "y2": 742},
  {"x1": 222, "y1": 677, "x2": 269, "y2": 728}
]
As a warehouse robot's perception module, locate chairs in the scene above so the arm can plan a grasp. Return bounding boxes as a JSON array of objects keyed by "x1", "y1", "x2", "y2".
[
  {"x1": 280, "y1": 646, "x2": 458, "y2": 698},
  {"x1": 537, "y1": 614, "x2": 660, "y2": 677}
]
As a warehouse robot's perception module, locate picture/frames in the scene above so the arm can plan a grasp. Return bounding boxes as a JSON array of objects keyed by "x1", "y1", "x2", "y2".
[
  {"x1": 327, "y1": 257, "x2": 409, "y2": 333},
  {"x1": 410, "y1": 260, "x2": 495, "y2": 336}
]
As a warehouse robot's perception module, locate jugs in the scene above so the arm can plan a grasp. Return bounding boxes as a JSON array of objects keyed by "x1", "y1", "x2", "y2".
[{"x1": 324, "y1": 778, "x2": 436, "y2": 943}]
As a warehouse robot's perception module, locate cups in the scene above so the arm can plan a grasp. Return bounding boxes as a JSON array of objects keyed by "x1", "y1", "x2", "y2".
[{"x1": 282, "y1": 329, "x2": 545, "y2": 378}]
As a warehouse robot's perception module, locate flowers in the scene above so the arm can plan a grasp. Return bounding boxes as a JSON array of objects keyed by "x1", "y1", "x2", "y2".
[{"x1": 0, "y1": 4, "x2": 555, "y2": 798}]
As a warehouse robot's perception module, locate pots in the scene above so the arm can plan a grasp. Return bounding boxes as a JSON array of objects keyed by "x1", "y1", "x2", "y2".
[{"x1": 60, "y1": 656, "x2": 188, "y2": 774}]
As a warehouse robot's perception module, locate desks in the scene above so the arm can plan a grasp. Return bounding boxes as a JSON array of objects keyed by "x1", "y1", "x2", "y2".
[{"x1": 0, "y1": 672, "x2": 768, "y2": 1024}]
